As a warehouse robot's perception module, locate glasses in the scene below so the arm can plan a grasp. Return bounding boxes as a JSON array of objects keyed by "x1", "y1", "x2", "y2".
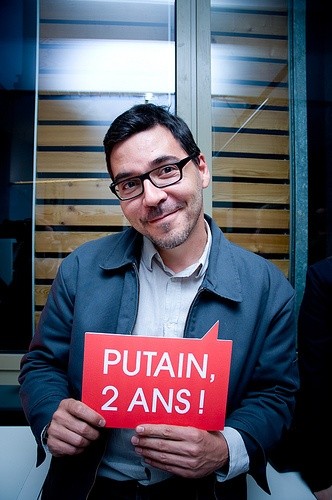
[{"x1": 109, "y1": 151, "x2": 200, "y2": 201}]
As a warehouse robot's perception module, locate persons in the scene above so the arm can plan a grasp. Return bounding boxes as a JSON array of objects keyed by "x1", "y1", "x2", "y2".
[
  {"x1": 285, "y1": 256, "x2": 332, "y2": 500},
  {"x1": 17, "y1": 103, "x2": 303, "y2": 500}
]
